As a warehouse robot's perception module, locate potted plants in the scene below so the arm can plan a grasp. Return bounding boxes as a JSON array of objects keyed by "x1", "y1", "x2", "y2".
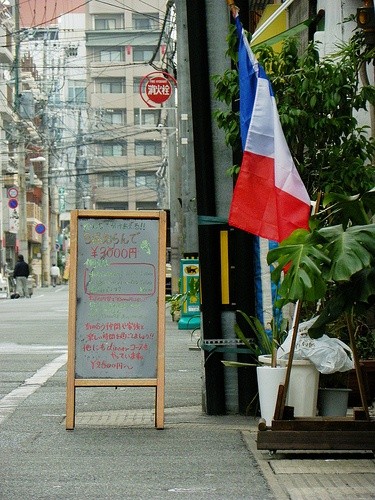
[{"x1": 212, "y1": 12, "x2": 375, "y2": 426}]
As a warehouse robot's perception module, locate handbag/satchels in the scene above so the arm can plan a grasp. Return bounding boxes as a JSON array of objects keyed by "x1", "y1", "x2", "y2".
[{"x1": 276, "y1": 315, "x2": 356, "y2": 374}]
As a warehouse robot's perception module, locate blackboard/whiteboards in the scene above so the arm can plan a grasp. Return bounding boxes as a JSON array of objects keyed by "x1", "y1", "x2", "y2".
[{"x1": 64, "y1": 209, "x2": 167, "y2": 429}]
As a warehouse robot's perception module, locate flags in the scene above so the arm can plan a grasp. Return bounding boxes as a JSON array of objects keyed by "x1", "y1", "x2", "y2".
[{"x1": 228, "y1": 16, "x2": 311, "y2": 279}]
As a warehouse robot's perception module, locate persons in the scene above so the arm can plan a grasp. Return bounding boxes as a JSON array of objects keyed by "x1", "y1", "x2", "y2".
[
  {"x1": 50, "y1": 263, "x2": 61, "y2": 287},
  {"x1": 13, "y1": 255, "x2": 30, "y2": 298}
]
